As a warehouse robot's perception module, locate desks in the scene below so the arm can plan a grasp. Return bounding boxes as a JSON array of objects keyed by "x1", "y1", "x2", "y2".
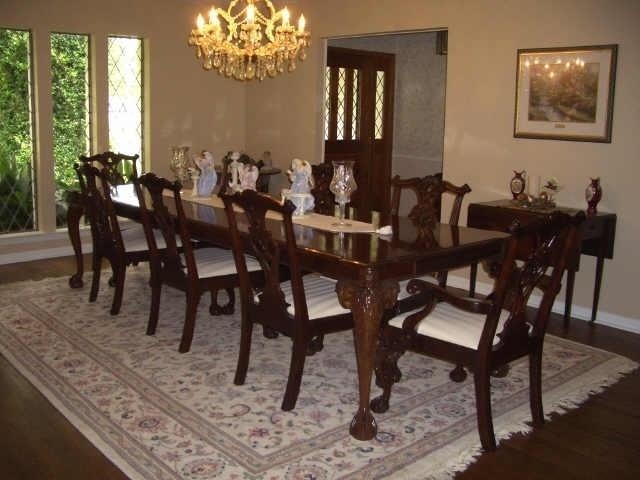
[
  {"x1": 465, "y1": 196, "x2": 618, "y2": 327},
  {"x1": 65, "y1": 183, "x2": 513, "y2": 441}
]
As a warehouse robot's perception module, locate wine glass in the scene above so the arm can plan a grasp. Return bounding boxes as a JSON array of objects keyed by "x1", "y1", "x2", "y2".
[
  {"x1": 329, "y1": 161, "x2": 358, "y2": 227},
  {"x1": 170, "y1": 145, "x2": 189, "y2": 197}
]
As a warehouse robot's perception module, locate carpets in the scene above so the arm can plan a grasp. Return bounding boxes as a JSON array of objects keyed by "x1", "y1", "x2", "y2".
[{"x1": 0, "y1": 262, "x2": 640, "y2": 480}]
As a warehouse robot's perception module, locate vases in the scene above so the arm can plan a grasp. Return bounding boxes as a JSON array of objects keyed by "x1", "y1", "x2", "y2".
[{"x1": 585, "y1": 177, "x2": 603, "y2": 214}]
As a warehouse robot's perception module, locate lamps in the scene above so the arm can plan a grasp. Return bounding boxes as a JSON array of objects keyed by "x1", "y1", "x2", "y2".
[{"x1": 188, "y1": 0, "x2": 311, "y2": 83}]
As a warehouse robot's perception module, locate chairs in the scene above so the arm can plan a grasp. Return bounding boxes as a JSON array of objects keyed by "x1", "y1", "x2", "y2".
[
  {"x1": 308, "y1": 161, "x2": 473, "y2": 313},
  {"x1": 128, "y1": 171, "x2": 278, "y2": 354},
  {"x1": 367, "y1": 210, "x2": 585, "y2": 451},
  {"x1": 74, "y1": 149, "x2": 198, "y2": 319},
  {"x1": 220, "y1": 189, "x2": 400, "y2": 412}
]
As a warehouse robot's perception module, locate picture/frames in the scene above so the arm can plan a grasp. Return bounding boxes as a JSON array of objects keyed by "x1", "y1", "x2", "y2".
[{"x1": 513, "y1": 43, "x2": 619, "y2": 143}]
